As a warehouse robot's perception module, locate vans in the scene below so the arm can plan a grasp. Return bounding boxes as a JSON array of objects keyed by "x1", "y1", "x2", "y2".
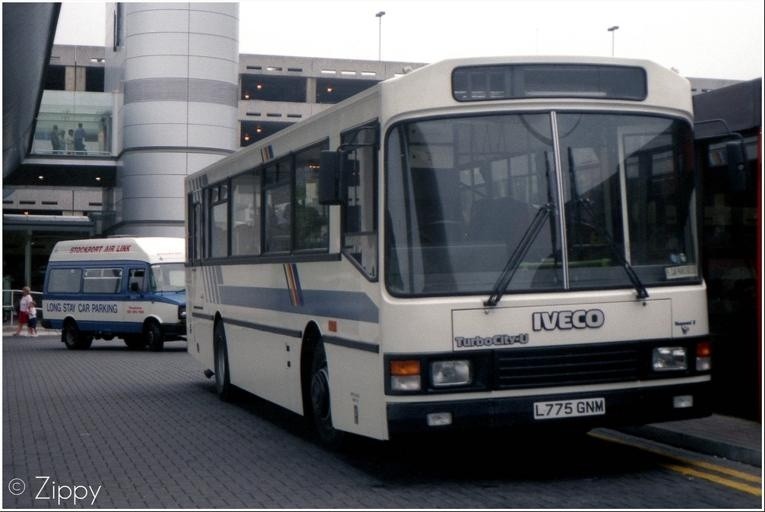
[{"x1": 40, "y1": 235, "x2": 190, "y2": 353}]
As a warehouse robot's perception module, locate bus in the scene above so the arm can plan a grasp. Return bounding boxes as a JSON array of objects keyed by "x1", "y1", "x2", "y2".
[{"x1": 182, "y1": 53, "x2": 751, "y2": 459}]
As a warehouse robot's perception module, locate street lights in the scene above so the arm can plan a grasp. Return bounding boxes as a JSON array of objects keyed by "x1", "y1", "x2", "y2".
[
  {"x1": 607, "y1": 25, "x2": 623, "y2": 57},
  {"x1": 375, "y1": 11, "x2": 386, "y2": 60}
]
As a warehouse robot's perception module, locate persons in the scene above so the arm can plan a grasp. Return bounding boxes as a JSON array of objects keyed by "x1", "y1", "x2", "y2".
[
  {"x1": 51, "y1": 119, "x2": 89, "y2": 155},
  {"x1": 13, "y1": 285, "x2": 31, "y2": 337},
  {"x1": 27, "y1": 300, "x2": 39, "y2": 337}
]
